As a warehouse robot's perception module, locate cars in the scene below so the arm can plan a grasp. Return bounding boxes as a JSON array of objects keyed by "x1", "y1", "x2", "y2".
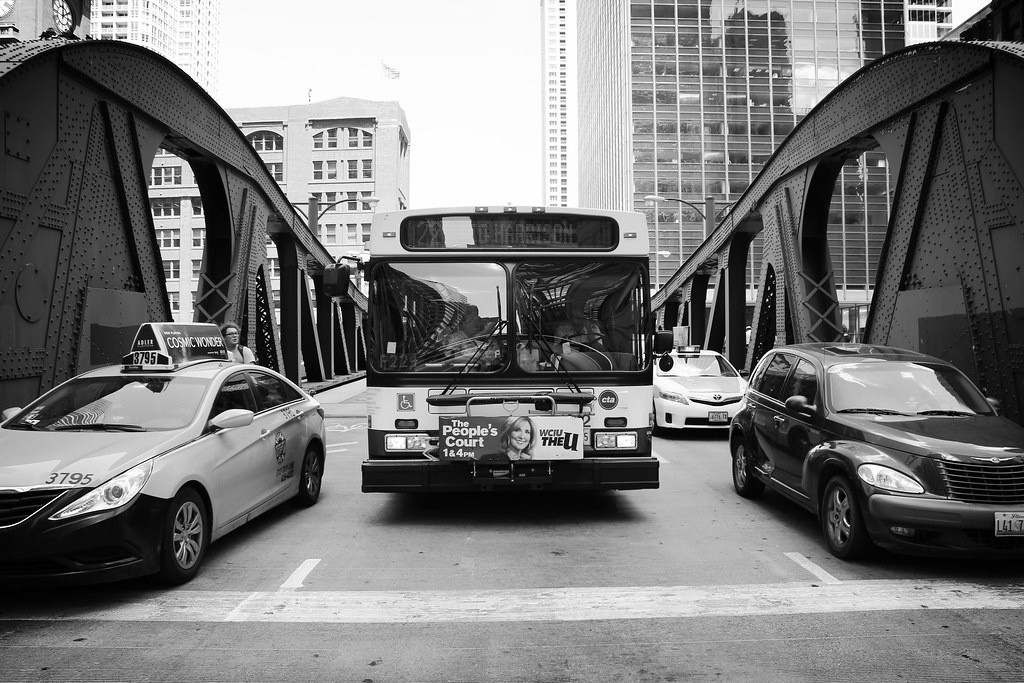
[
  {"x1": 728, "y1": 341, "x2": 1024, "y2": 565},
  {"x1": 651, "y1": 344, "x2": 751, "y2": 436},
  {"x1": 0, "y1": 321, "x2": 327, "y2": 588}
]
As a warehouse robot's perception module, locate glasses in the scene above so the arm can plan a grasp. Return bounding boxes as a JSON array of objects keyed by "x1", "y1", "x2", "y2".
[{"x1": 226, "y1": 332, "x2": 238, "y2": 337}]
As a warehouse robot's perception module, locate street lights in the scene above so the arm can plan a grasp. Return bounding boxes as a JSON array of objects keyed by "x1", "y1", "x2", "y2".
[
  {"x1": 292, "y1": 195, "x2": 381, "y2": 237},
  {"x1": 643, "y1": 194, "x2": 737, "y2": 242}
]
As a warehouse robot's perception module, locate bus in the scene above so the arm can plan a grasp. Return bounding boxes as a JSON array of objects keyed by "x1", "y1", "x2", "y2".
[{"x1": 319, "y1": 203, "x2": 675, "y2": 496}]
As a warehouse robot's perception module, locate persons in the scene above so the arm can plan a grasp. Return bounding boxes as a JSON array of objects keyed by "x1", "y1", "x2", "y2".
[
  {"x1": 219, "y1": 322, "x2": 256, "y2": 365},
  {"x1": 445, "y1": 307, "x2": 501, "y2": 365},
  {"x1": 479, "y1": 416, "x2": 536, "y2": 460}
]
